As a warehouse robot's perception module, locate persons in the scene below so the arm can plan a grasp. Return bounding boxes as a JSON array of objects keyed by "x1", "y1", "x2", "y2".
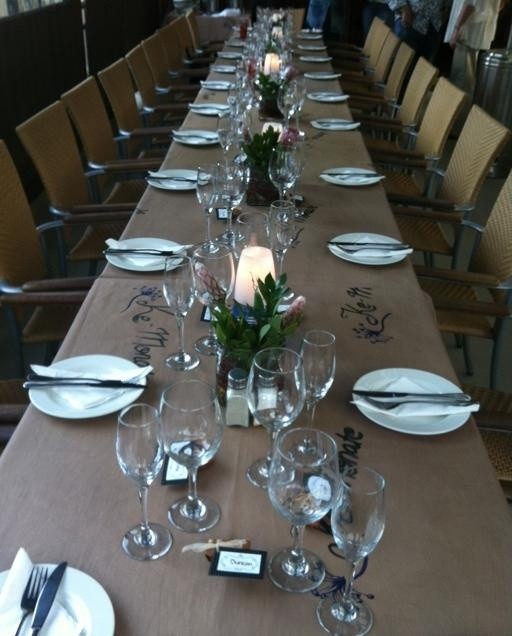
[
  {"x1": 385, "y1": 0, "x2": 453, "y2": 66},
  {"x1": 448, "y1": 0, "x2": 507, "y2": 138}
]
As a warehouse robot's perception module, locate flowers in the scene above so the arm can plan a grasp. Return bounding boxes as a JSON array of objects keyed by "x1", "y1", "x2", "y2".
[
  {"x1": 235, "y1": 124, "x2": 299, "y2": 177},
  {"x1": 271, "y1": 15, "x2": 288, "y2": 26},
  {"x1": 249, "y1": 65, "x2": 299, "y2": 103},
  {"x1": 192, "y1": 260, "x2": 306, "y2": 366}
]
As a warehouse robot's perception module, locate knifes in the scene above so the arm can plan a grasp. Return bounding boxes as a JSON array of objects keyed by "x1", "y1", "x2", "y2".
[
  {"x1": 349, "y1": 386, "x2": 473, "y2": 404},
  {"x1": 22, "y1": 381, "x2": 148, "y2": 391},
  {"x1": 30, "y1": 561, "x2": 69, "y2": 636},
  {"x1": 327, "y1": 237, "x2": 408, "y2": 247},
  {"x1": 147, "y1": 175, "x2": 198, "y2": 183}
]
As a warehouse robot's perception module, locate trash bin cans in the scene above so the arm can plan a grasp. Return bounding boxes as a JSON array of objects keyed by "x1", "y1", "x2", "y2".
[{"x1": 472, "y1": 49, "x2": 512, "y2": 179}]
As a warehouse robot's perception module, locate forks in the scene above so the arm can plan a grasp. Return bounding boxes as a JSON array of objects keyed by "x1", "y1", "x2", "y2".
[
  {"x1": 358, "y1": 393, "x2": 475, "y2": 412},
  {"x1": 191, "y1": 105, "x2": 230, "y2": 112},
  {"x1": 173, "y1": 134, "x2": 219, "y2": 140},
  {"x1": 10, "y1": 566, "x2": 50, "y2": 636},
  {"x1": 26, "y1": 366, "x2": 154, "y2": 385},
  {"x1": 337, "y1": 242, "x2": 410, "y2": 253},
  {"x1": 102, "y1": 246, "x2": 186, "y2": 257}
]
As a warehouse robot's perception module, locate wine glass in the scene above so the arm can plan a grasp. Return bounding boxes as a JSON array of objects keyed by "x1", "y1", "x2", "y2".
[
  {"x1": 300, "y1": 329, "x2": 337, "y2": 430},
  {"x1": 266, "y1": 426, "x2": 341, "y2": 594},
  {"x1": 233, "y1": 211, "x2": 272, "y2": 263},
  {"x1": 316, "y1": 465, "x2": 387, "y2": 636},
  {"x1": 267, "y1": 144, "x2": 305, "y2": 218},
  {"x1": 161, "y1": 255, "x2": 202, "y2": 373},
  {"x1": 246, "y1": 346, "x2": 306, "y2": 489},
  {"x1": 115, "y1": 402, "x2": 173, "y2": 562},
  {"x1": 268, "y1": 200, "x2": 295, "y2": 301},
  {"x1": 196, "y1": 162, "x2": 220, "y2": 254},
  {"x1": 192, "y1": 244, "x2": 236, "y2": 356},
  {"x1": 213, "y1": 160, "x2": 247, "y2": 243},
  {"x1": 210, "y1": 4, "x2": 305, "y2": 164},
  {"x1": 159, "y1": 379, "x2": 225, "y2": 534}
]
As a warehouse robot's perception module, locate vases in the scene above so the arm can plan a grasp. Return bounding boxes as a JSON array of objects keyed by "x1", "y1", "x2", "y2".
[
  {"x1": 213, "y1": 339, "x2": 284, "y2": 408},
  {"x1": 243, "y1": 164, "x2": 279, "y2": 206},
  {"x1": 255, "y1": 93, "x2": 285, "y2": 121}
]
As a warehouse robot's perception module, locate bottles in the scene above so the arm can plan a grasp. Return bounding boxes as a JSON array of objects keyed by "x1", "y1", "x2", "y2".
[{"x1": 222, "y1": 368, "x2": 250, "y2": 430}]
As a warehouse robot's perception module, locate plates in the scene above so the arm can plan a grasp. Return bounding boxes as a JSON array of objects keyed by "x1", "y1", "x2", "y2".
[
  {"x1": 22, "y1": 353, "x2": 156, "y2": 421},
  {"x1": 325, "y1": 231, "x2": 415, "y2": 267},
  {"x1": 101, "y1": 237, "x2": 195, "y2": 273},
  {"x1": 144, "y1": 167, "x2": 213, "y2": 192},
  {"x1": 201, "y1": 80, "x2": 236, "y2": 91},
  {"x1": 0, "y1": 560, "x2": 116, "y2": 636},
  {"x1": 189, "y1": 103, "x2": 230, "y2": 116},
  {"x1": 297, "y1": 28, "x2": 388, "y2": 188},
  {"x1": 347, "y1": 362, "x2": 483, "y2": 437},
  {"x1": 172, "y1": 128, "x2": 220, "y2": 146}
]
